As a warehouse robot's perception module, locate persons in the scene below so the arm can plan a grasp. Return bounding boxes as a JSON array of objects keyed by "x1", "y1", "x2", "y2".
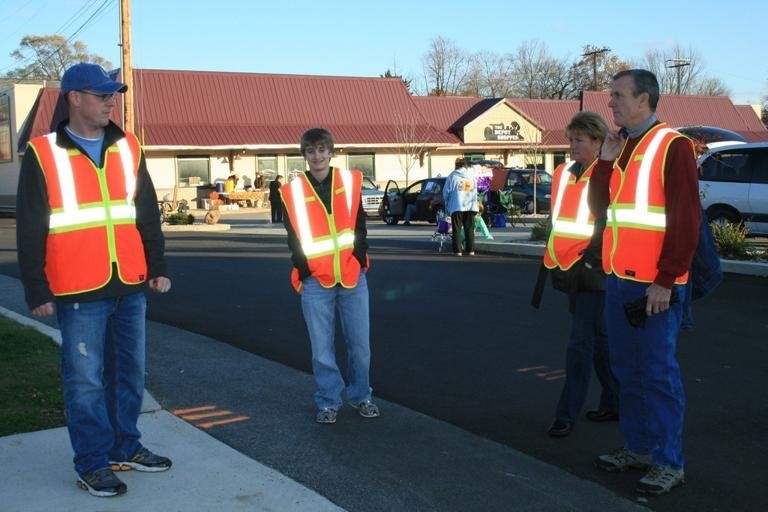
[
  {"x1": 443, "y1": 156, "x2": 477, "y2": 256},
  {"x1": 228, "y1": 173, "x2": 240, "y2": 193},
  {"x1": 270, "y1": 175, "x2": 284, "y2": 223},
  {"x1": 278, "y1": 128, "x2": 381, "y2": 426},
  {"x1": 597, "y1": 71, "x2": 702, "y2": 495},
  {"x1": 14, "y1": 61, "x2": 173, "y2": 499},
  {"x1": 404, "y1": 204, "x2": 416, "y2": 225},
  {"x1": 254, "y1": 171, "x2": 267, "y2": 190},
  {"x1": 545, "y1": 111, "x2": 610, "y2": 437}
]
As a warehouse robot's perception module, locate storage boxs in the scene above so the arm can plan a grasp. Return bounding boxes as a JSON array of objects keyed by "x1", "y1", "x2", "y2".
[{"x1": 215, "y1": 181, "x2": 225, "y2": 192}]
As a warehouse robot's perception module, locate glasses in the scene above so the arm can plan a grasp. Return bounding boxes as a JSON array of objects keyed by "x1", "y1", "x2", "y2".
[{"x1": 73, "y1": 89, "x2": 116, "y2": 103}]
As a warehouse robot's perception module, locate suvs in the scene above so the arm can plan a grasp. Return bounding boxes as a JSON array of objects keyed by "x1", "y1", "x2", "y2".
[
  {"x1": 695, "y1": 141, "x2": 767, "y2": 247},
  {"x1": 360, "y1": 175, "x2": 391, "y2": 219}
]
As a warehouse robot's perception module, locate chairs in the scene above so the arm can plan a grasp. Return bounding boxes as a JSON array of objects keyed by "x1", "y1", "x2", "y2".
[
  {"x1": 157, "y1": 186, "x2": 180, "y2": 225},
  {"x1": 496, "y1": 187, "x2": 530, "y2": 231}
]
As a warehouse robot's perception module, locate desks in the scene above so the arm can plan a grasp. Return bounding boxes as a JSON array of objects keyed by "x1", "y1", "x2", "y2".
[
  {"x1": 201, "y1": 198, "x2": 217, "y2": 210},
  {"x1": 218, "y1": 191, "x2": 264, "y2": 212}
]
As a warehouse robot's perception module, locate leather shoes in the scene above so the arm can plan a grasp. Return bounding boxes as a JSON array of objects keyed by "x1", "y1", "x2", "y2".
[
  {"x1": 548, "y1": 417, "x2": 574, "y2": 436},
  {"x1": 586, "y1": 408, "x2": 619, "y2": 423}
]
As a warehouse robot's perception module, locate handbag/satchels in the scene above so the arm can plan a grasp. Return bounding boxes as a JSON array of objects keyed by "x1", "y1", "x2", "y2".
[{"x1": 692, "y1": 208, "x2": 723, "y2": 297}]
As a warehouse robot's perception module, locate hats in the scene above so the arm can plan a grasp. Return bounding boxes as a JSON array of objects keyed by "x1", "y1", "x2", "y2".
[{"x1": 61, "y1": 62, "x2": 128, "y2": 95}]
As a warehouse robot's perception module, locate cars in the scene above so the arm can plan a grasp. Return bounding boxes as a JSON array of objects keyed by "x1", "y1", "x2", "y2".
[
  {"x1": 495, "y1": 168, "x2": 554, "y2": 215},
  {"x1": 377, "y1": 176, "x2": 448, "y2": 227}
]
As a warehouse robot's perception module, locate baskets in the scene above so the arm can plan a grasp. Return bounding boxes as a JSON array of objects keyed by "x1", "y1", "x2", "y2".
[{"x1": 492, "y1": 213, "x2": 506, "y2": 227}]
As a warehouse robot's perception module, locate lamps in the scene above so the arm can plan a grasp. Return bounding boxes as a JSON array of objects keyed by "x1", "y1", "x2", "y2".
[
  {"x1": 234, "y1": 149, "x2": 241, "y2": 162},
  {"x1": 332, "y1": 150, "x2": 337, "y2": 158},
  {"x1": 241, "y1": 149, "x2": 246, "y2": 155},
  {"x1": 339, "y1": 148, "x2": 344, "y2": 153}
]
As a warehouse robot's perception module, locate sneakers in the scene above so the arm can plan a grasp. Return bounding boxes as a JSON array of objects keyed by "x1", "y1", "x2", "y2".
[
  {"x1": 594, "y1": 446, "x2": 648, "y2": 473},
  {"x1": 348, "y1": 400, "x2": 380, "y2": 418},
  {"x1": 315, "y1": 408, "x2": 338, "y2": 423},
  {"x1": 76, "y1": 467, "x2": 127, "y2": 497},
  {"x1": 108, "y1": 446, "x2": 172, "y2": 472},
  {"x1": 632, "y1": 463, "x2": 687, "y2": 496}
]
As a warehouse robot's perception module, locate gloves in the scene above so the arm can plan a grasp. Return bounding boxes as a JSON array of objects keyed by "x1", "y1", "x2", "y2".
[{"x1": 624, "y1": 285, "x2": 680, "y2": 328}]
